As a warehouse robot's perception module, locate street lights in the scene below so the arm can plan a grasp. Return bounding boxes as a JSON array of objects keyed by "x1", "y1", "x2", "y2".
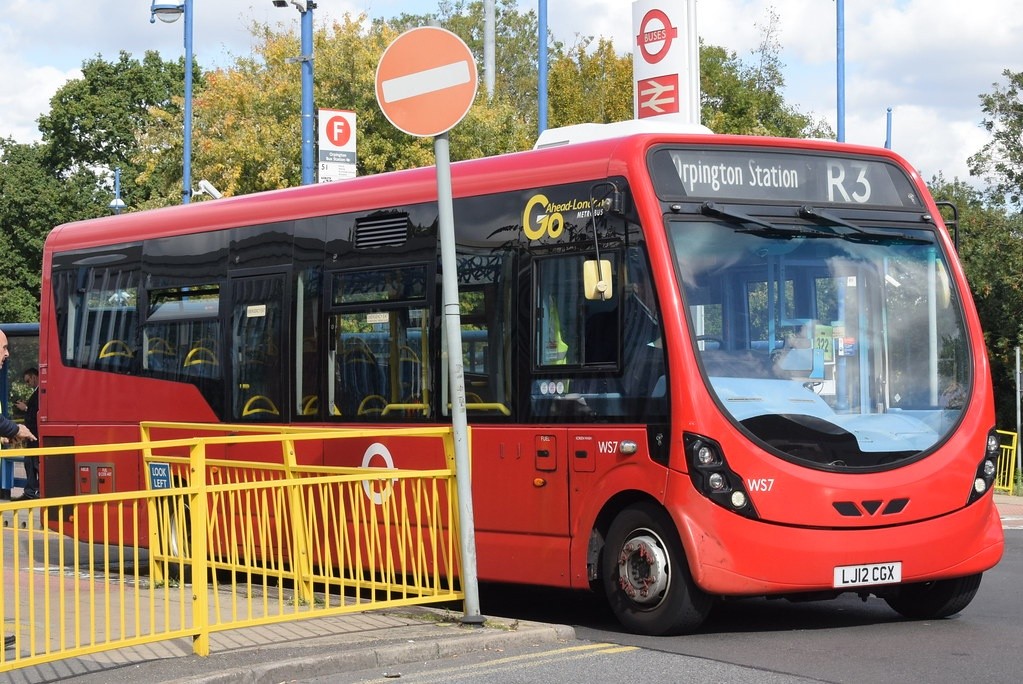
[{"x1": 148, "y1": 0, "x2": 194, "y2": 206}]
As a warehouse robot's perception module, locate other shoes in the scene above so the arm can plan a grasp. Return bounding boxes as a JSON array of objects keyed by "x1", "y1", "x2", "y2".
[{"x1": 10, "y1": 493, "x2": 32, "y2": 501}]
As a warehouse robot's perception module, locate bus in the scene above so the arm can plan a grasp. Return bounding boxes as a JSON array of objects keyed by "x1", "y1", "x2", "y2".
[
  {"x1": 332, "y1": 329, "x2": 490, "y2": 417},
  {"x1": 40, "y1": 120, "x2": 1008, "y2": 638}
]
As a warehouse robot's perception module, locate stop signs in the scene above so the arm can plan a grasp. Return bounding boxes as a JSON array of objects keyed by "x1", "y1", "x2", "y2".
[{"x1": 375, "y1": 26, "x2": 478, "y2": 138}]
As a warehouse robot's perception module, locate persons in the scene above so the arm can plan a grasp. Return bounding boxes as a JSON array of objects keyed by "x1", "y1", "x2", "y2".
[
  {"x1": 11, "y1": 368, "x2": 40, "y2": 501},
  {"x1": 0, "y1": 330, "x2": 37, "y2": 647},
  {"x1": 604, "y1": 272, "x2": 662, "y2": 360}
]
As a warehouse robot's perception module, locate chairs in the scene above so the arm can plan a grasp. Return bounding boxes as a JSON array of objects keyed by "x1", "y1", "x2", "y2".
[{"x1": 98, "y1": 336, "x2": 487, "y2": 417}]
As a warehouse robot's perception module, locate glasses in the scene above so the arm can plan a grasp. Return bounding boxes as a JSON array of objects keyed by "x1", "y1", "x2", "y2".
[{"x1": 26, "y1": 376, "x2": 31, "y2": 383}]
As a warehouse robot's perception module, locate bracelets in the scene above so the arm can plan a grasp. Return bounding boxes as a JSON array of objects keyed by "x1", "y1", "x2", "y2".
[{"x1": 24, "y1": 406, "x2": 28, "y2": 412}]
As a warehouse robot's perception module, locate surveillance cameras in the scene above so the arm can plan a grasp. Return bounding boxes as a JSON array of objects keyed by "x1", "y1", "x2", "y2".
[{"x1": 198, "y1": 179, "x2": 223, "y2": 199}]
